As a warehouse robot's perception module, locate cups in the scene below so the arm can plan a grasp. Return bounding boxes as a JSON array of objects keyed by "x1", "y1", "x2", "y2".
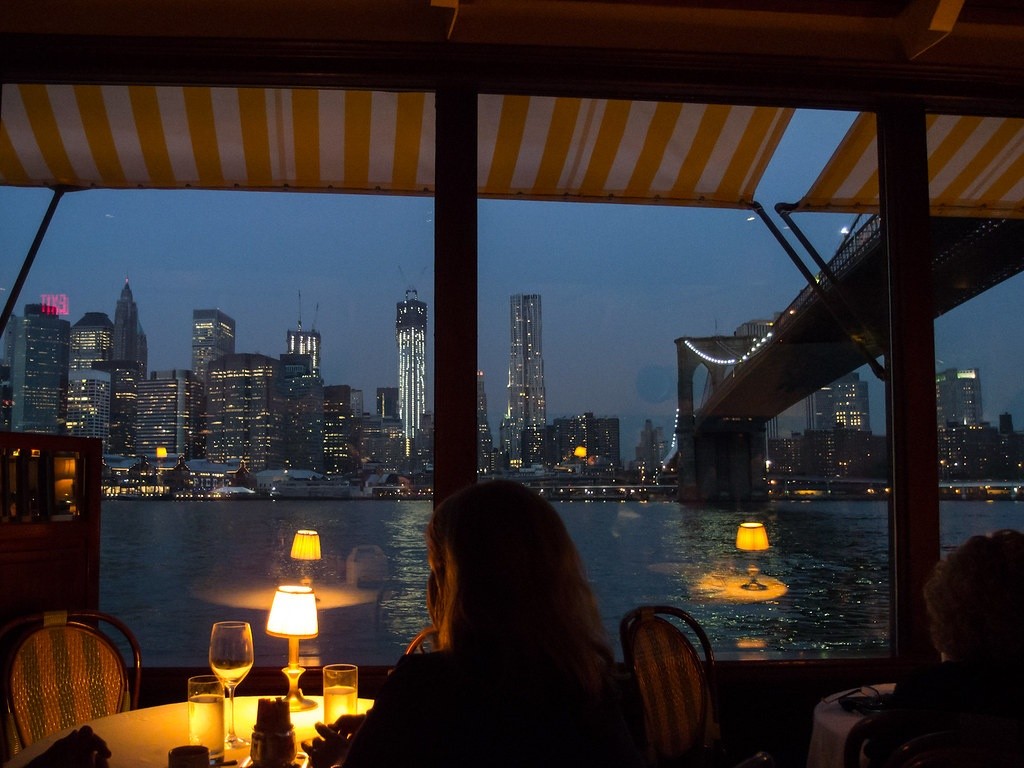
[
  {"x1": 322, "y1": 663, "x2": 358, "y2": 726},
  {"x1": 168, "y1": 745, "x2": 209, "y2": 768},
  {"x1": 187, "y1": 675, "x2": 225, "y2": 766}
]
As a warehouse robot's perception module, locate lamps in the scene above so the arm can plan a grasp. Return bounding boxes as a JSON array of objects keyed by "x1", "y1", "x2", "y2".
[
  {"x1": 289, "y1": 529, "x2": 322, "y2": 600},
  {"x1": 265, "y1": 585, "x2": 319, "y2": 712},
  {"x1": 736, "y1": 522, "x2": 770, "y2": 591}
]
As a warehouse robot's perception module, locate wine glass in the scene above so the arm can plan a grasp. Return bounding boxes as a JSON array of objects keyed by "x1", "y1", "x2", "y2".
[{"x1": 208, "y1": 621, "x2": 255, "y2": 749}]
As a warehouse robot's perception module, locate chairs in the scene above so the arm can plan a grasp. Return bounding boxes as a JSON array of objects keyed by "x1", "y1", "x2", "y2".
[
  {"x1": 404, "y1": 626, "x2": 438, "y2": 654},
  {"x1": 0, "y1": 609, "x2": 143, "y2": 763},
  {"x1": 843, "y1": 709, "x2": 1024, "y2": 768},
  {"x1": 618, "y1": 604, "x2": 721, "y2": 768}
]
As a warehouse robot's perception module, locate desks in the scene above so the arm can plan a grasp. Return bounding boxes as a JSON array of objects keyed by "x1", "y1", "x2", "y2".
[
  {"x1": 806, "y1": 682, "x2": 896, "y2": 768},
  {"x1": 5, "y1": 696, "x2": 375, "y2": 768}
]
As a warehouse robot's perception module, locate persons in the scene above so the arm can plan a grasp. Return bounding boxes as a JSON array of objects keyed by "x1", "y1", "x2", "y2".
[
  {"x1": 316, "y1": 544, "x2": 389, "y2": 667},
  {"x1": 301, "y1": 480, "x2": 614, "y2": 768},
  {"x1": 864, "y1": 528, "x2": 1024, "y2": 760}
]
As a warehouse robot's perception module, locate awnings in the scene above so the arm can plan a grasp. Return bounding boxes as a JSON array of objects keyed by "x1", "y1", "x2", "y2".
[
  {"x1": 0, "y1": 83, "x2": 887, "y2": 381},
  {"x1": 774, "y1": 112, "x2": 1024, "y2": 356}
]
